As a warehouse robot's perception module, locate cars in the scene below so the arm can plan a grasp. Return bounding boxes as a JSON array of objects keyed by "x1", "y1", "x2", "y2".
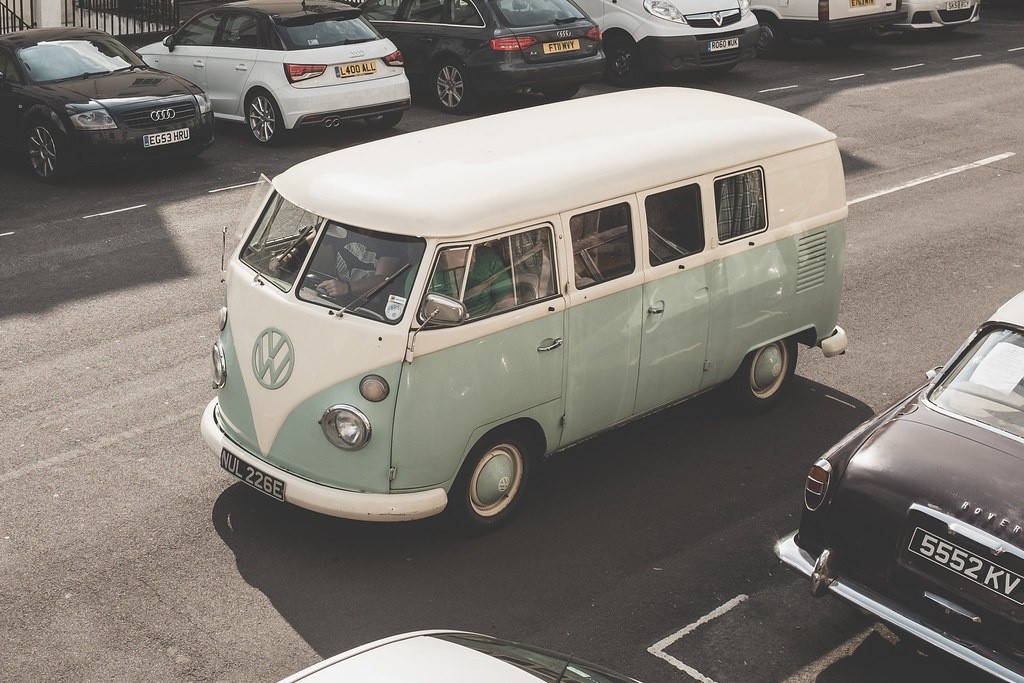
[
  {"x1": 894, "y1": 0, "x2": 981, "y2": 35},
  {"x1": 276, "y1": 629, "x2": 642, "y2": 683},
  {"x1": 0, "y1": 27, "x2": 215, "y2": 184},
  {"x1": 772, "y1": 290, "x2": 1024, "y2": 683}
]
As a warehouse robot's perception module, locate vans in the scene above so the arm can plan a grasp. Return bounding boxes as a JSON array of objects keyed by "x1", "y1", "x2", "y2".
[
  {"x1": 197, "y1": 88, "x2": 848, "y2": 533},
  {"x1": 498, "y1": 0, "x2": 761, "y2": 87},
  {"x1": 747, "y1": 0, "x2": 903, "y2": 57}
]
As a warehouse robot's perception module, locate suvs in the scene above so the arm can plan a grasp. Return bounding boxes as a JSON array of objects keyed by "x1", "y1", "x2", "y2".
[
  {"x1": 353, "y1": 0, "x2": 607, "y2": 115},
  {"x1": 133, "y1": 0, "x2": 412, "y2": 148}
]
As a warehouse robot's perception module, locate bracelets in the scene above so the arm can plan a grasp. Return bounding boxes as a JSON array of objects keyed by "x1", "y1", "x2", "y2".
[{"x1": 345, "y1": 280, "x2": 353, "y2": 294}]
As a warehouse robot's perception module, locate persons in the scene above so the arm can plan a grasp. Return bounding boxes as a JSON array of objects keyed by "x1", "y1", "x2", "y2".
[
  {"x1": 404, "y1": 238, "x2": 517, "y2": 319},
  {"x1": 268, "y1": 222, "x2": 406, "y2": 303}
]
